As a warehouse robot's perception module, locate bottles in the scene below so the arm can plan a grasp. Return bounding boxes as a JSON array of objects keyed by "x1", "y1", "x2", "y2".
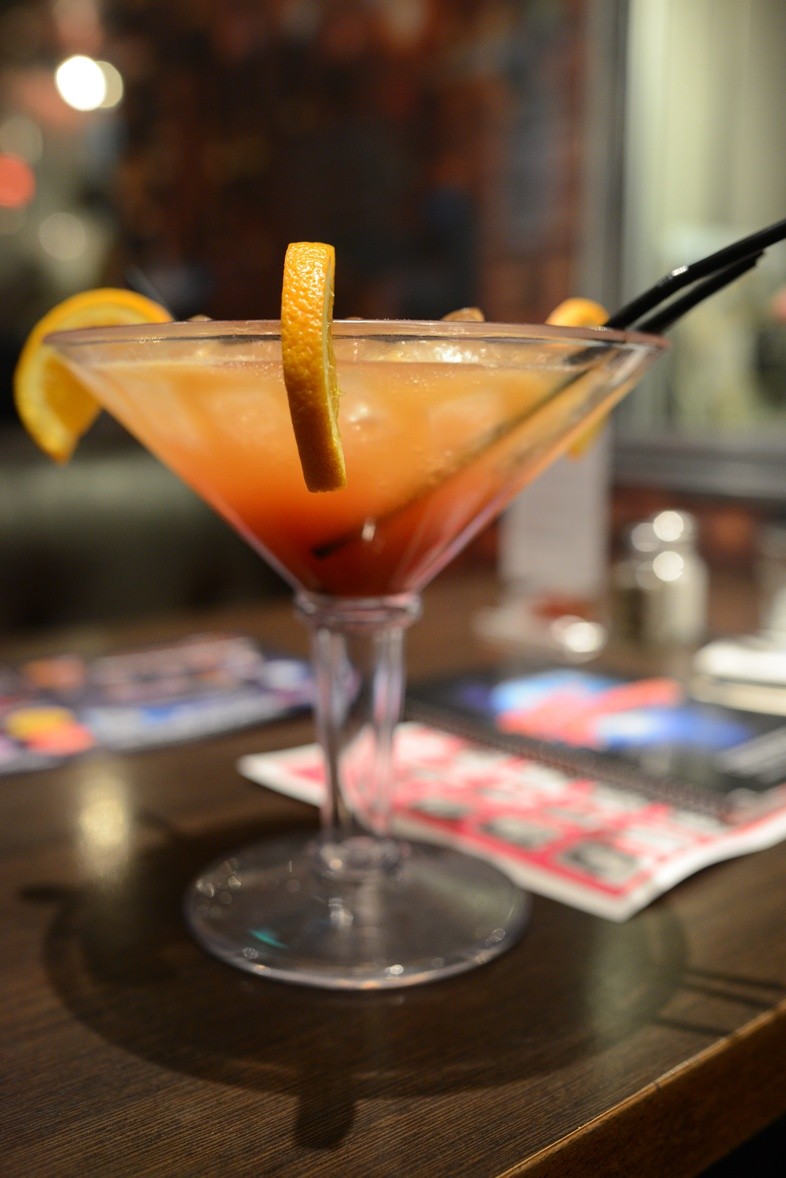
[{"x1": 610, "y1": 508, "x2": 708, "y2": 656}]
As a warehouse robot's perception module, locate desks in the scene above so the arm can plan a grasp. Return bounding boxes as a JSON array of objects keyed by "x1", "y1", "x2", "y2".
[{"x1": 1, "y1": 564, "x2": 786, "y2": 1178}]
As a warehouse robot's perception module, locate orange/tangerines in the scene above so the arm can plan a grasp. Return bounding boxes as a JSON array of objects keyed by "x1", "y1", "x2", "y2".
[
  {"x1": 279, "y1": 241, "x2": 348, "y2": 493},
  {"x1": 547, "y1": 297, "x2": 611, "y2": 465},
  {"x1": 16, "y1": 288, "x2": 181, "y2": 460}
]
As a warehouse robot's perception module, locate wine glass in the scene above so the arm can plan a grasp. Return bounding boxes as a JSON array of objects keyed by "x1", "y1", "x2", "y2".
[{"x1": 42, "y1": 319, "x2": 671, "y2": 989}]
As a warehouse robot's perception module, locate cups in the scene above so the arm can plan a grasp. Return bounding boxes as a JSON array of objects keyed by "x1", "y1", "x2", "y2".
[{"x1": 490, "y1": 408, "x2": 609, "y2": 646}]
